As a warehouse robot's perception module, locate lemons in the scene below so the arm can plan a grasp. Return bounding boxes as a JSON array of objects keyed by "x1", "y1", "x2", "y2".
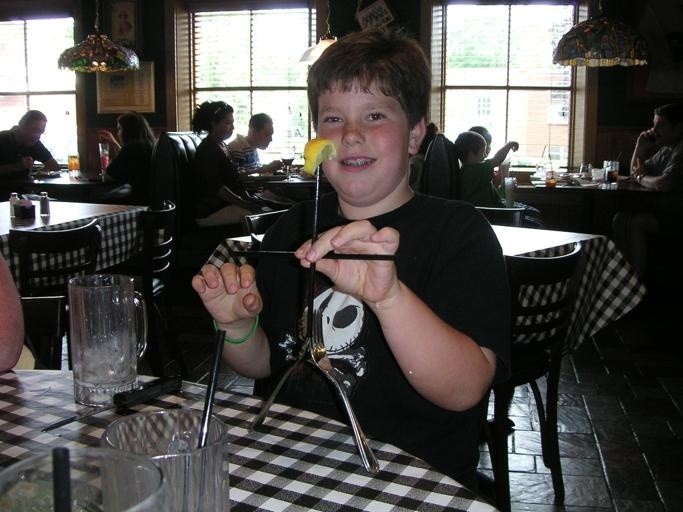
[{"x1": 303, "y1": 138, "x2": 337, "y2": 175}]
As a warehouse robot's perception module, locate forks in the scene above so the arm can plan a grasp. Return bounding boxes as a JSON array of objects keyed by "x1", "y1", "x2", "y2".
[
  {"x1": 310, "y1": 309, "x2": 380, "y2": 474},
  {"x1": 250, "y1": 310, "x2": 323, "y2": 430}
]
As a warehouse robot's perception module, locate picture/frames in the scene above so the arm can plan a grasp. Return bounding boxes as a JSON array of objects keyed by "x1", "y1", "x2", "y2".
[
  {"x1": 94, "y1": 60, "x2": 157, "y2": 116},
  {"x1": 101, "y1": 2, "x2": 141, "y2": 57}
]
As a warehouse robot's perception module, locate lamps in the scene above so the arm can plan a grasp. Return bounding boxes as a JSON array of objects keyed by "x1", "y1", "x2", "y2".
[
  {"x1": 56, "y1": 3, "x2": 140, "y2": 76},
  {"x1": 550, "y1": 3, "x2": 651, "y2": 68}
]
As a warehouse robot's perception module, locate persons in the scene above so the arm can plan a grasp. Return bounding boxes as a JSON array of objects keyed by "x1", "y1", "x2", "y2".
[
  {"x1": 454, "y1": 132, "x2": 539, "y2": 230},
  {"x1": 0, "y1": 109, "x2": 60, "y2": 202},
  {"x1": 229, "y1": 114, "x2": 282, "y2": 182},
  {"x1": 190, "y1": 26, "x2": 507, "y2": 487},
  {"x1": 184, "y1": 101, "x2": 266, "y2": 226},
  {"x1": 615, "y1": 106, "x2": 683, "y2": 241},
  {"x1": 468, "y1": 125, "x2": 508, "y2": 190},
  {"x1": 98, "y1": 109, "x2": 158, "y2": 205}
]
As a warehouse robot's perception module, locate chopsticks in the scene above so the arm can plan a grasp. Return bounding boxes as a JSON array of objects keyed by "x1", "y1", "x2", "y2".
[{"x1": 230, "y1": 163, "x2": 398, "y2": 337}]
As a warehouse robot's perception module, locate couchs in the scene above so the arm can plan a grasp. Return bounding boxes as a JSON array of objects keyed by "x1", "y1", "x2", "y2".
[
  {"x1": 419, "y1": 133, "x2": 527, "y2": 228},
  {"x1": 147, "y1": 129, "x2": 253, "y2": 245}
]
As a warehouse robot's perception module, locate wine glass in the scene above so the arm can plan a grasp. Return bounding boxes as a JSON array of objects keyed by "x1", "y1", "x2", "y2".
[{"x1": 281, "y1": 146, "x2": 294, "y2": 177}]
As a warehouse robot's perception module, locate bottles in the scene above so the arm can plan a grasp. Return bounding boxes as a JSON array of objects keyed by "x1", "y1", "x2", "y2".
[
  {"x1": 580, "y1": 163, "x2": 586, "y2": 173},
  {"x1": 588, "y1": 164, "x2": 592, "y2": 174},
  {"x1": 40, "y1": 192, "x2": 49, "y2": 214},
  {"x1": 10, "y1": 193, "x2": 20, "y2": 216}
]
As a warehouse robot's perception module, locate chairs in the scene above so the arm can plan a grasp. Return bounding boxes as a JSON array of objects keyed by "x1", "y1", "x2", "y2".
[
  {"x1": 489, "y1": 239, "x2": 590, "y2": 512},
  {"x1": 129, "y1": 199, "x2": 186, "y2": 368},
  {"x1": 7, "y1": 218, "x2": 104, "y2": 370}
]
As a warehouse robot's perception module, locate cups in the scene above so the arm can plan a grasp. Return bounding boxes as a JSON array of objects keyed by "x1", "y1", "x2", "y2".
[
  {"x1": 545, "y1": 160, "x2": 557, "y2": 188},
  {"x1": 499, "y1": 160, "x2": 509, "y2": 182},
  {"x1": 68, "y1": 151, "x2": 79, "y2": 177},
  {"x1": 0, "y1": 448, "x2": 166, "y2": 511},
  {"x1": 603, "y1": 160, "x2": 618, "y2": 189},
  {"x1": 99, "y1": 143, "x2": 110, "y2": 175},
  {"x1": 69, "y1": 274, "x2": 148, "y2": 407},
  {"x1": 505, "y1": 177, "x2": 515, "y2": 208},
  {"x1": 580, "y1": 163, "x2": 590, "y2": 177},
  {"x1": 102, "y1": 410, "x2": 230, "y2": 512}
]
